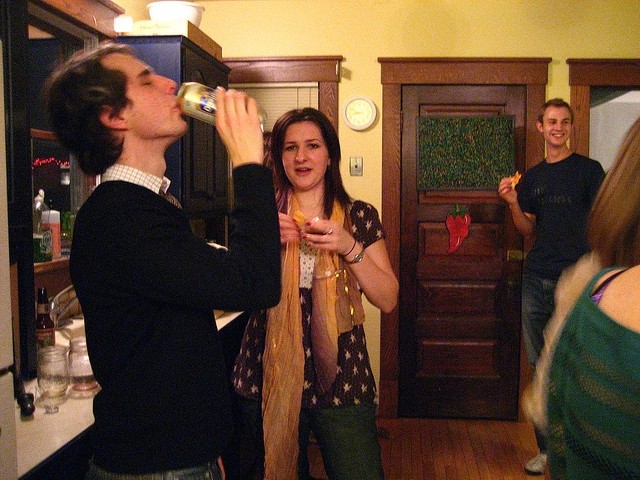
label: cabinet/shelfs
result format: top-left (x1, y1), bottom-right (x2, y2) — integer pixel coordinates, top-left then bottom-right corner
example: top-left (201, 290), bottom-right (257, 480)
top-left (116, 35), bottom-right (232, 216)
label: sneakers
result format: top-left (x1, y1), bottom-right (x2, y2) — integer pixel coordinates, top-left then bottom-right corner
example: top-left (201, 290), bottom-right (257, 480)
top-left (524, 453), bottom-right (547, 476)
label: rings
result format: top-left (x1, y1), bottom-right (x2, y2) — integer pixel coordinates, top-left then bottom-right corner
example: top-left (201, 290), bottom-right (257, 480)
top-left (327, 227), bottom-right (332, 234)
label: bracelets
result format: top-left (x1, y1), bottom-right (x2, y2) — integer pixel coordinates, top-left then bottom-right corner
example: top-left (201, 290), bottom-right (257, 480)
top-left (343, 239), bottom-right (358, 256)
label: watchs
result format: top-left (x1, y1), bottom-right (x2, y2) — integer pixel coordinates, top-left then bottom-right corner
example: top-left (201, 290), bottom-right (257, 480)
top-left (344, 246), bottom-right (366, 262)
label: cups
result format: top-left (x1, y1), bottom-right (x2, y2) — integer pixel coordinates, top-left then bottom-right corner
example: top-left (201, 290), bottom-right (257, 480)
top-left (68, 341), bottom-right (100, 400)
top-left (33, 190), bottom-right (53, 262)
top-left (36, 345), bottom-right (69, 407)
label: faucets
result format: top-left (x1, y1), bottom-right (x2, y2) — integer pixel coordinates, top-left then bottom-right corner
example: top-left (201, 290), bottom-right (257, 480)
top-left (49, 284), bottom-right (77, 327)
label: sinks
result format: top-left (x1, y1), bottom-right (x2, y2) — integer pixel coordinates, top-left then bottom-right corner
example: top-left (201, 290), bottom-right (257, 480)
top-left (54, 314), bottom-right (87, 347)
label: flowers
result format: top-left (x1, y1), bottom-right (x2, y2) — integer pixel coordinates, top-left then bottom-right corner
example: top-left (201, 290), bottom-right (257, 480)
top-left (31, 156), bottom-right (71, 192)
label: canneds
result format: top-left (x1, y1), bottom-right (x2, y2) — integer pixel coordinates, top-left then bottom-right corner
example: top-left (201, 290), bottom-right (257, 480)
top-left (176, 81), bottom-right (268, 134)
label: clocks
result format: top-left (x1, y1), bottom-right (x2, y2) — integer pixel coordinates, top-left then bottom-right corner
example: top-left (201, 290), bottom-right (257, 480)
top-left (343, 94), bottom-right (377, 131)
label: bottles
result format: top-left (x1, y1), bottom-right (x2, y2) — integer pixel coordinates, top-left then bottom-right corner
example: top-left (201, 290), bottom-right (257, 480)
top-left (31, 287), bottom-right (54, 384)
top-left (42, 210), bottom-right (61, 258)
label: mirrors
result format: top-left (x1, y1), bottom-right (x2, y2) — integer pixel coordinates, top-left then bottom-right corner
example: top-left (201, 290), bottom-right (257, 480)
top-left (13, 0), bottom-right (96, 274)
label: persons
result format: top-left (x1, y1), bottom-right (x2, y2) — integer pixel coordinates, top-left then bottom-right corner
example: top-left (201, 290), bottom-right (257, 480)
top-left (232, 108), bottom-right (400, 479)
top-left (544, 118), bottom-right (639, 479)
top-left (43, 41), bottom-right (283, 480)
top-left (497, 97), bottom-right (606, 475)
